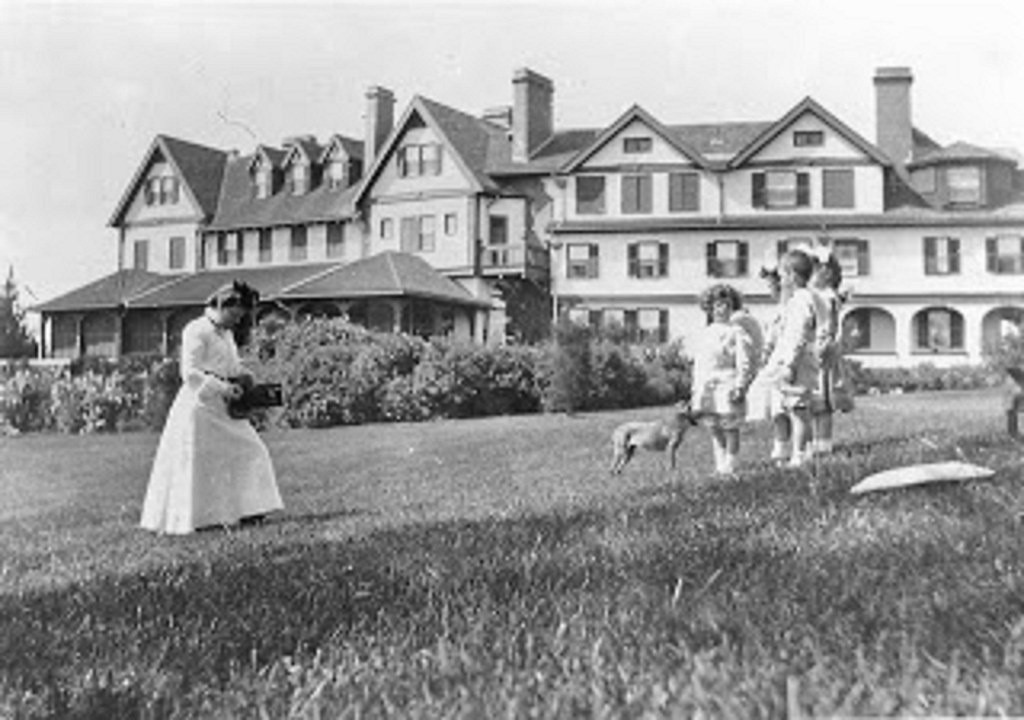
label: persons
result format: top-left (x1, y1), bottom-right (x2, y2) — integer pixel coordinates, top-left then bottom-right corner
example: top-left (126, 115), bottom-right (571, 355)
top-left (140, 275), bottom-right (287, 537)
top-left (690, 249), bottom-right (853, 475)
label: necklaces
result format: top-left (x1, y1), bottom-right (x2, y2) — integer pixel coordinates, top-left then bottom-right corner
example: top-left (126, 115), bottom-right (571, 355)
top-left (206, 316), bottom-right (223, 330)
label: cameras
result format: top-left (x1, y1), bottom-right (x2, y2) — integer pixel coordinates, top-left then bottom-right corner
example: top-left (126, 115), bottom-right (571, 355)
top-left (228, 374), bottom-right (283, 419)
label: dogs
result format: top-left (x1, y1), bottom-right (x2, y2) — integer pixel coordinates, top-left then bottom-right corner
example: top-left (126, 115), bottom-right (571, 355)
top-left (604, 405), bottom-right (698, 477)
